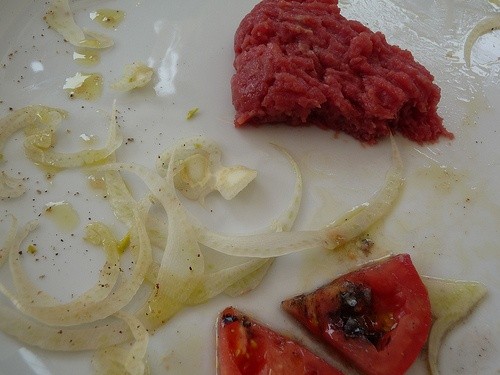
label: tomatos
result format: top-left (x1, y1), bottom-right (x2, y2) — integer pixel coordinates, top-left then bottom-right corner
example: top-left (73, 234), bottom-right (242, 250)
top-left (216, 254), bottom-right (433, 375)
